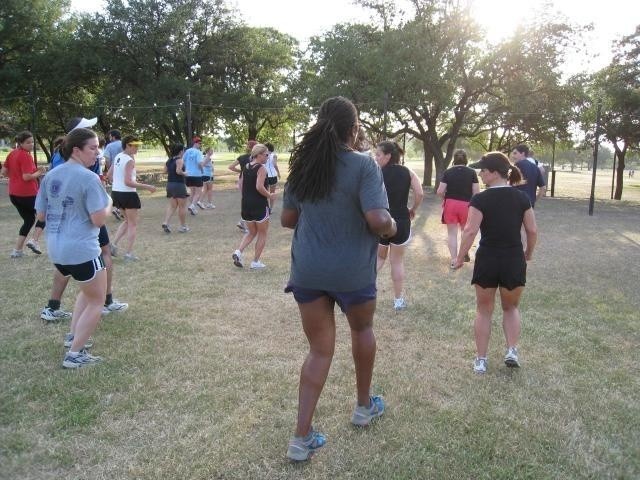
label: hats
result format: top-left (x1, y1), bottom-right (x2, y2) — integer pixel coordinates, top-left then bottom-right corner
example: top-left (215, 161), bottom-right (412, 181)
top-left (67, 117), bottom-right (97, 134)
top-left (127, 140), bottom-right (144, 145)
top-left (251, 144), bottom-right (268, 158)
top-left (468, 154), bottom-right (509, 172)
top-left (193, 136), bottom-right (201, 142)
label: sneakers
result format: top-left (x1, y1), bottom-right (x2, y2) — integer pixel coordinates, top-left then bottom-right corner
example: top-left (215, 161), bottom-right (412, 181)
top-left (110, 242), bottom-right (119, 257)
top-left (237, 221), bottom-right (249, 233)
top-left (393, 297), bottom-right (407, 310)
top-left (62, 332), bottom-right (102, 368)
top-left (350, 395), bottom-right (384, 426)
top-left (177, 224), bottom-right (190, 232)
top-left (122, 251), bottom-right (139, 261)
top-left (162, 223), bottom-right (171, 232)
top-left (250, 259), bottom-right (266, 269)
top-left (10, 248), bottom-right (28, 258)
top-left (40, 305), bottom-right (72, 320)
top-left (451, 252), bottom-right (470, 269)
top-left (26, 238), bottom-right (42, 254)
top-left (188, 200), bottom-right (216, 215)
top-left (504, 347), bottom-right (520, 367)
top-left (232, 249), bottom-right (244, 268)
top-left (112, 208), bottom-right (124, 220)
top-left (472, 355), bottom-right (487, 374)
top-left (102, 302), bottom-right (128, 313)
top-left (286, 426), bottom-right (326, 461)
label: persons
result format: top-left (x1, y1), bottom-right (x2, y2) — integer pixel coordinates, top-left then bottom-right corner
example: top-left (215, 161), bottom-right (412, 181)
top-left (284, 122), bottom-right (367, 294)
top-left (163, 140), bottom-right (280, 268)
top-left (279, 95), bottom-right (395, 461)
top-left (4, 122), bottom-right (157, 366)
top-left (373, 141), bottom-right (423, 308)
top-left (509, 145), bottom-right (545, 223)
top-left (435, 150), bottom-right (481, 267)
top-left (528, 149), bottom-right (545, 174)
top-left (454, 152), bottom-right (537, 373)
